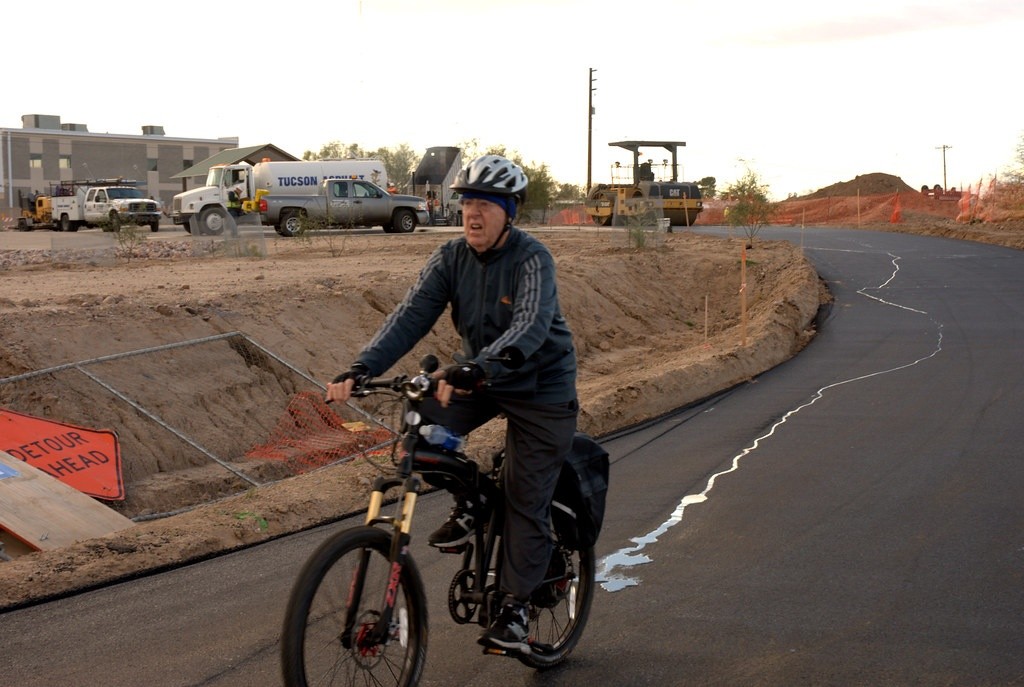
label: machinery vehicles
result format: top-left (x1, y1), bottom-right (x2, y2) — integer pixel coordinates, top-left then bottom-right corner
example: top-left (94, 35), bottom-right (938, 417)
top-left (19, 189), bottom-right (52, 230)
top-left (587, 140), bottom-right (704, 227)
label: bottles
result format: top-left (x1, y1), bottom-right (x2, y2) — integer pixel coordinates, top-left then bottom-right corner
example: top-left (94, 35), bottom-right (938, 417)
top-left (419, 424), bottom-right (465, 451)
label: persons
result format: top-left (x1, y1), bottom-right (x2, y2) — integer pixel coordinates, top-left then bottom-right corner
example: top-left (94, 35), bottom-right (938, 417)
top-left (324, 153), bottom-right (580, 656)
top-left (226, 184), bottom-right (250, 238)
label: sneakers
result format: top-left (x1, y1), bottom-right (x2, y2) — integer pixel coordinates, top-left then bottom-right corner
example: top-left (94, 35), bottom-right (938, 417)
top-left (426, 502), bottom-right (490, 547)
top-left (477, 602), bottom-right (530, 649)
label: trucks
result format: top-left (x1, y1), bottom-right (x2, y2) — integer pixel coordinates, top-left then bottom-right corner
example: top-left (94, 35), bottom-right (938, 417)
top-left (168, 157), bottom-right (388, 236)
top-left (52, 177), bottom-right (163, 232)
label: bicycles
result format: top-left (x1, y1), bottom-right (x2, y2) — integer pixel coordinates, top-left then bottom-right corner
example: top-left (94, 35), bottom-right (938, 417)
top-left (281, 353), bottom-right (595, 687)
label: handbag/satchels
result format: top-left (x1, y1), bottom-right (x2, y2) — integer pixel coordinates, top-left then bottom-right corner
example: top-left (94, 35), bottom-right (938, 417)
top-left (549, 437), bottom-right (609, 551)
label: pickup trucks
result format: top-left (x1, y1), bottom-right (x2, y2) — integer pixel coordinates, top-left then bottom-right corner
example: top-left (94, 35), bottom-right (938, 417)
top-left (259, 176), bottom-right (430, 237)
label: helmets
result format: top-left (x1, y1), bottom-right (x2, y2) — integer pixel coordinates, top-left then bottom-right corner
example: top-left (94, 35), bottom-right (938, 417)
top-left (449, 155), bottom-right (529, 206)
